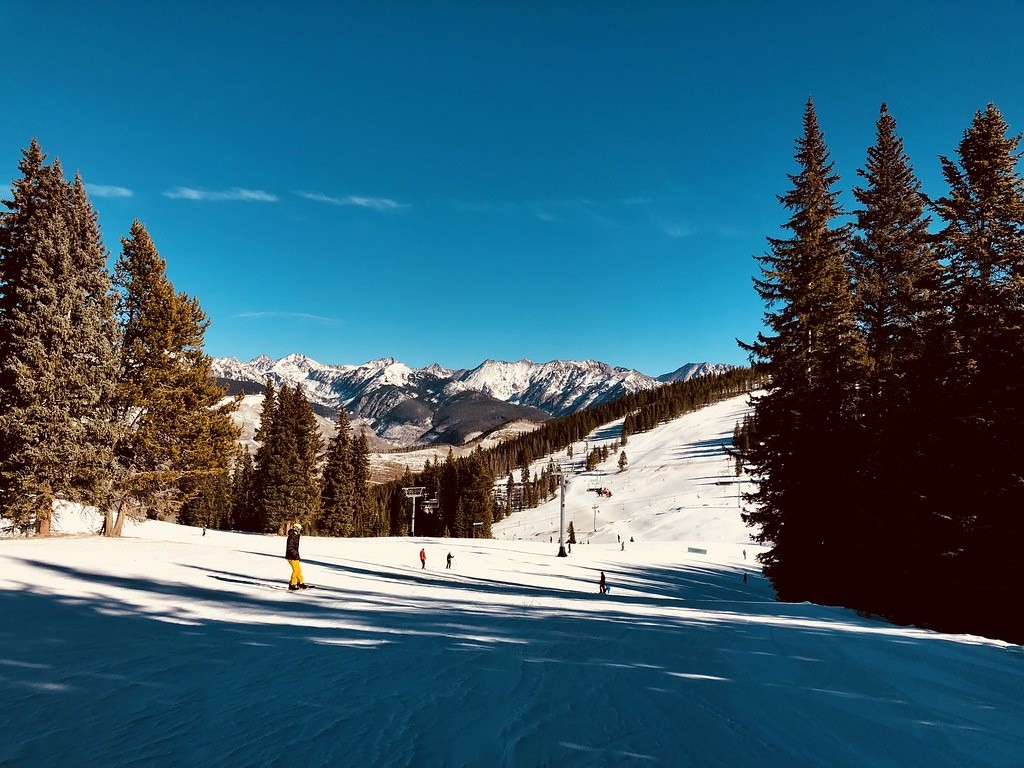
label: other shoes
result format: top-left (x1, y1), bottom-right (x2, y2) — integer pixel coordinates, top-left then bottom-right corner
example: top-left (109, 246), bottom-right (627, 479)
top-left (297, 583), bottom-right (307, 589)
top-left (289, 585), bottom-right (299, 590)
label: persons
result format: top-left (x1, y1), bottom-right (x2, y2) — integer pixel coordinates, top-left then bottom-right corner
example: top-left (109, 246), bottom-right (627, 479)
top-left (742, 573), bottom-right (748, 583)
top-left (549, 536), bottom-right (553, 544)
top-left (617, 534), bottom-right (620, 543)
top-left (597, 487), bottom-right (613, 498)
top-left (446, 551), bottom-right (454, 569)
top-left (597, 571), bottom-right (606, 595)
top-left (742, 549), bottom-right (748, 560)
top-left (621, 541), bottom-right (624, 551)
top-left (567, 542), bottom-right (571, 553)
top-left (419, 547), bottom-right (427, 571)
top-left (284, 523), bottom-right (311, 592)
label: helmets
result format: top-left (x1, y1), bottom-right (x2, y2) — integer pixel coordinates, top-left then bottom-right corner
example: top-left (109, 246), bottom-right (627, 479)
top-left (293, 523), bottom-right (304, 532)
